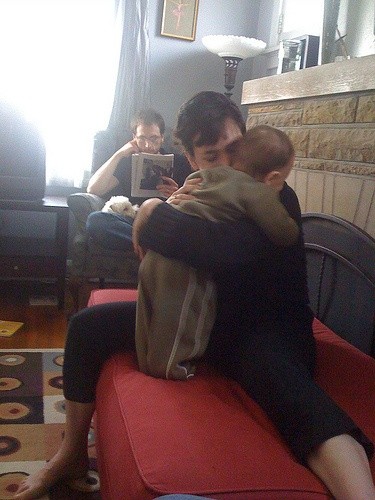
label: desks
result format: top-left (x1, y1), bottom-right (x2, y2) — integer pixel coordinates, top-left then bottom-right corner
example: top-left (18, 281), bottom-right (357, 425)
top-left (0, 197), bottom-right (70, 312)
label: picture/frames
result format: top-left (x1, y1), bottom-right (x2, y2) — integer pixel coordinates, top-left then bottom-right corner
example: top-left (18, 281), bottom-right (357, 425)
top-left (160, 0), bottom-right (199, 41)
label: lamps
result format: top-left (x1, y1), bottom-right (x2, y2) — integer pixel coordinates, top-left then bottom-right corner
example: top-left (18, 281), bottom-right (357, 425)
top-left (202, 34), bottom-right (267, 100)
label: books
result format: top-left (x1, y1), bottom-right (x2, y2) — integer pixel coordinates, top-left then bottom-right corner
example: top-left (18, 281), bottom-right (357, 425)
top-left (132, 153), bottom-right (174, 197)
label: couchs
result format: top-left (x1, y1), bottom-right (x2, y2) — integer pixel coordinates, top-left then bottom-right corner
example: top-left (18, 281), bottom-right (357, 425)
top-left (0, 104), bottom-right (46, 200)
top-left (68, 127), bottom-right (181, 289)
top-left (88, 212), bottom-right (375, 500)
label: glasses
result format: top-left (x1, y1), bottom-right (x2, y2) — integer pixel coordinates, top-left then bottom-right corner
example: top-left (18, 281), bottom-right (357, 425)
top-left (135, 135), bottom-right (161, 142)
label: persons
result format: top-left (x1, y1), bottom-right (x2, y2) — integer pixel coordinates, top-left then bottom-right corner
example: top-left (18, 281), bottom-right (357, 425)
top-left (135, 124), bottom-right (299, 380)
top-left (86, 108), bottom-right (192, 253)
top-left (171, 0), bottom-right (192, 30)
top-left (14, 92), bottom-right (375, 500)
